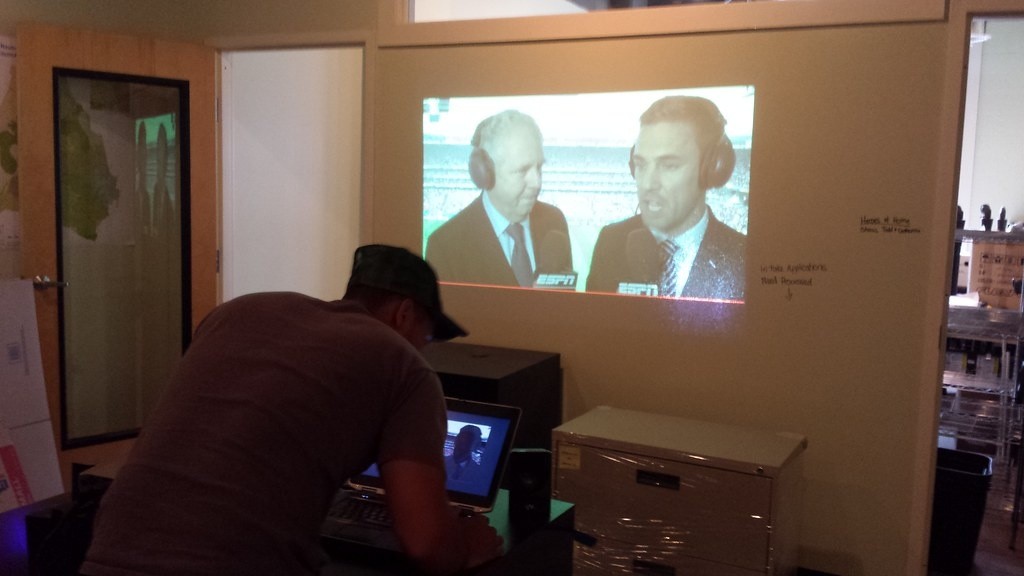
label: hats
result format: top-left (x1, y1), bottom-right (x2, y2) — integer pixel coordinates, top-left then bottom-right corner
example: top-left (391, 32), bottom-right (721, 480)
top-left (354, 244), bottom-right (468, 340)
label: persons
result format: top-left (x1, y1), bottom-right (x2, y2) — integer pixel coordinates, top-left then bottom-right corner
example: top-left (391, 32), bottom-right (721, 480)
top-left (445, 425), bottom-right (482, 492)
top-left (78, 243), bottom-right (505, 576)
top-left (426, 110), bottom-right (572, 288)
top-left (587, 95), bottom-right (744, 302)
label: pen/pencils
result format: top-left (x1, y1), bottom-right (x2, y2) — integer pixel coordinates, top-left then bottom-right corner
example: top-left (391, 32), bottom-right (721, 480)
top-left (463, 509), bottom-right (478, 518)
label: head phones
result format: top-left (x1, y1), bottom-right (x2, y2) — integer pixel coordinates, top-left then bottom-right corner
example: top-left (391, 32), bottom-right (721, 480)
top-left (629, 122), bottom-right (736, 191)
top-left (469, 119), bottom-right (495, 191)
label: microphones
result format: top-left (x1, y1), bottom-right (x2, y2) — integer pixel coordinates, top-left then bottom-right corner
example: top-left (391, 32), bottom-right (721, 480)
top-left (617, 229), bottom-right (663, 296)
top-left (531, 230), bottom-right (578, 290)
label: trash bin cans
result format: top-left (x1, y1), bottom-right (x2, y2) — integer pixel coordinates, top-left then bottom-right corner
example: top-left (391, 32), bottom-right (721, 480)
top-left (928, 444), bottom-right (993, 575)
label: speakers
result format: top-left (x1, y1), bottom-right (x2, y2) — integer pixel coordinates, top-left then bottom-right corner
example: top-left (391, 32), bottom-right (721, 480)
top-left (509, 447), bottom-right (553, 533)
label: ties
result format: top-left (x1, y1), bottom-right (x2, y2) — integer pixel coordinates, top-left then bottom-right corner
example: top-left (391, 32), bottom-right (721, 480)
top-left (658, 239), bottom-right (681, 298)
top-left (506, 223), bottom-right (535, 287)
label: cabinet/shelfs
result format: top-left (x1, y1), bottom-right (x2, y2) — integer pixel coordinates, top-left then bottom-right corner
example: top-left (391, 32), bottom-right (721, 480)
top-left (938, 306), bottom-right (1024, 465)
top-left (551, 406), bottom-right (808, 576)
top-left (426, 342), bottom-right (559, 541)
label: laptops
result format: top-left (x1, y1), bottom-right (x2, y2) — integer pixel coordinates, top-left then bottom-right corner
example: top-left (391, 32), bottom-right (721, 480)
top-left (318, 397), bottom-right (523, 553)
